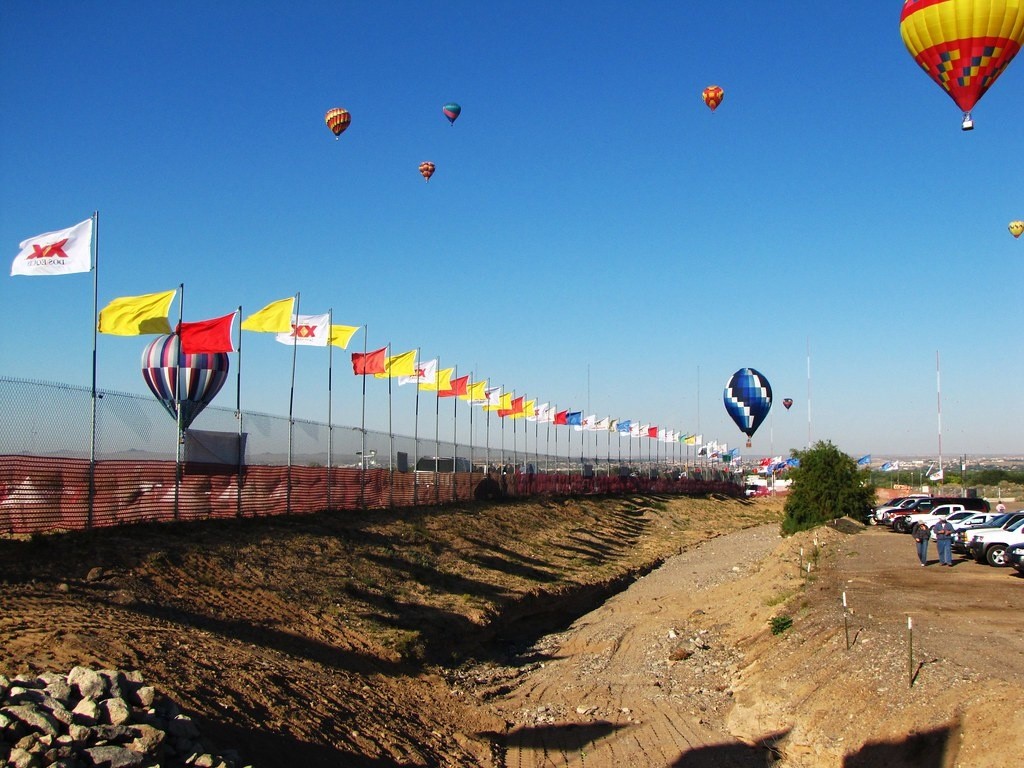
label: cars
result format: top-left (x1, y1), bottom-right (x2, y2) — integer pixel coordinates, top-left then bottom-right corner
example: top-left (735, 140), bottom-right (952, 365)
top-left (865, 494), bottom-right (929, 526)
top-left (930, 511), bottom-right (1024, 576)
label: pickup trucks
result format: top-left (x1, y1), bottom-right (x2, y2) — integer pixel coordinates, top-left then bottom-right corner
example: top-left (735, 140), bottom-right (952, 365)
top-left (904, 505), bottom-right (965, 532)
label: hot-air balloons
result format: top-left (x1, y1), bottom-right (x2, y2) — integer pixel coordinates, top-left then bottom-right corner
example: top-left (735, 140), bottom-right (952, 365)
top-left (899, 0), bottom-right (1024, 131)
top-left (702, 85), bottom-right (725, 114)
top-left (783, 398), bottom-right (793, 412)
top-left (1008, 220), bottom-right (1024, 240)
top-left (722, 367), bottom-right (773, 449)
top-left (325, 108), bottom-right (352, 141)
top-left (443, 103), bottom-right (461, 127)
top-left (419, 162), bottom-right (435, 184)
top-left (141, 332), bottom-right (230, 444)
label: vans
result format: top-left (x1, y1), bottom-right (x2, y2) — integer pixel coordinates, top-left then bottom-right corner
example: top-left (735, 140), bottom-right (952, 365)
top-left (412, 455), bottom-right (477, 487)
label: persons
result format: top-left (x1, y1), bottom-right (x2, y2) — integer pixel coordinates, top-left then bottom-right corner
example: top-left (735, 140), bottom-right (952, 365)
top-left (933, 516), bottom-right (955, 566)
top-left (995, 500), bottom-right (1005, 513)
top-left (911, 520), bottom-right (931, 567)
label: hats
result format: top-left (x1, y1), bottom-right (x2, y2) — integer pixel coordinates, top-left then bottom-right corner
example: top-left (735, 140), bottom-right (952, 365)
top-left (918, 520), bottom-right (924, 526)
top-left (940, 517), bottom-right (946, 521)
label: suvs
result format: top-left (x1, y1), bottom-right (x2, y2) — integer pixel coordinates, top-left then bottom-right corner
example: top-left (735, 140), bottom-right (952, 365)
top-left (882, 497), bottom-right (990, 532)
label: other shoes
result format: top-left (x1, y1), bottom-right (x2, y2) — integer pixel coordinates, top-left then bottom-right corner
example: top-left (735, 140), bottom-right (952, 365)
top-left (940, 562), bottom-right (944, 566)
top-left (948, 562), bottom-right (952, 566)
top-left (921, 563), bottom-right (925, 567)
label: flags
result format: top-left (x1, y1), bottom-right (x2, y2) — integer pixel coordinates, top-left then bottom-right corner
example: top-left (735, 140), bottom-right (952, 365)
top-left (274, 311), bottom-right (743, 468)
top-left (238, 297), bottom-right (296, 333)
top-left (175, 313), bottom-right (236, 355)
top-left (10, 218), bottom-right (92, 276)
top-left (97, 288), bottom-right (174, 334)
top-left (752, 456), bottom-right (801, 474)
top-left (881, 461), bottom-right (944, 481)
top-left (858, 455), bottom-right (871, 466)
